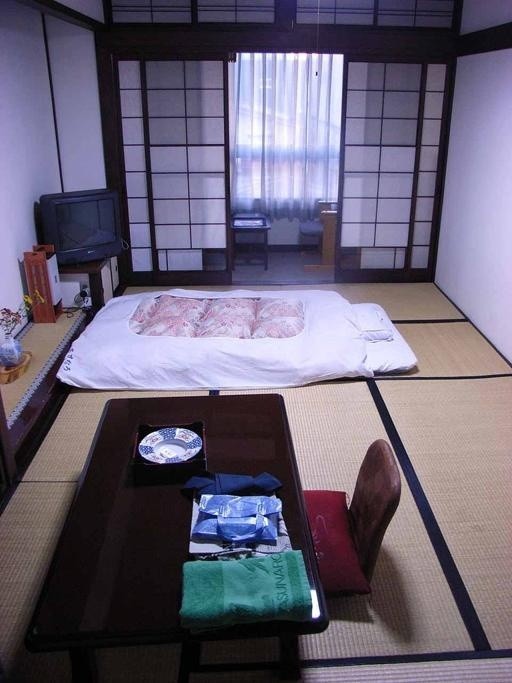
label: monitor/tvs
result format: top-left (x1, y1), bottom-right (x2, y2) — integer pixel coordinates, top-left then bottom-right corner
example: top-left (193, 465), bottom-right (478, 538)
top-left (39, 188), bottom-right (122, 266)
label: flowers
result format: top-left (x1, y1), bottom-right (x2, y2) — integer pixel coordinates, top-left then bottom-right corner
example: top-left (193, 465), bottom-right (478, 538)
top-left (1, 286), bottom-right (46, 335)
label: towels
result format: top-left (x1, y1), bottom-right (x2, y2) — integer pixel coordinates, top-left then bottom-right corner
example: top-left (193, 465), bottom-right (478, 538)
top-left (172, 542), bottom-right (313, 637)
top-left (178, 462), bottom-right (289, 500)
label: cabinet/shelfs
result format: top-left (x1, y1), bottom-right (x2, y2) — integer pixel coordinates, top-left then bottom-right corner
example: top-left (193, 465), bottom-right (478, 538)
top-left (54, 253), bottom-right (125, 327)
top-left (0, 303), bottom-right (97, 505)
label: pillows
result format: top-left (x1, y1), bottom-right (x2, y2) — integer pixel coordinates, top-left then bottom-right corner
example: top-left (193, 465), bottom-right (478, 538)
top-left (346, 300), bottom-right (396, 343)
top-left (302, 487), bottom-right (372, 599)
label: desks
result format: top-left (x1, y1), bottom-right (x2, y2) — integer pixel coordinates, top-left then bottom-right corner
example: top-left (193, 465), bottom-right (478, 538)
top-left (20, 386), bottom-right (333, 682)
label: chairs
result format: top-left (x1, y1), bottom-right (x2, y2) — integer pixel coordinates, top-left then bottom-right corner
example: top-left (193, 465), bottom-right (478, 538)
top-left (299, 435), bottom-right (403, 601)
top-left (297, 197), bottom-right (341, 274)
top-left (227, 207), bottom-right (273, 273)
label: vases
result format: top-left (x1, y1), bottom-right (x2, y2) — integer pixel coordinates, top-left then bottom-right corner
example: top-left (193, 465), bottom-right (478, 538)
top-left (0, 331), bottom-right (27, 369)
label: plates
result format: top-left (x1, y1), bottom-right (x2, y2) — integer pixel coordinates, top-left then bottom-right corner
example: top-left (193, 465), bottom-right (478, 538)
top-left (138, 427), bottom-right (202, 464)
